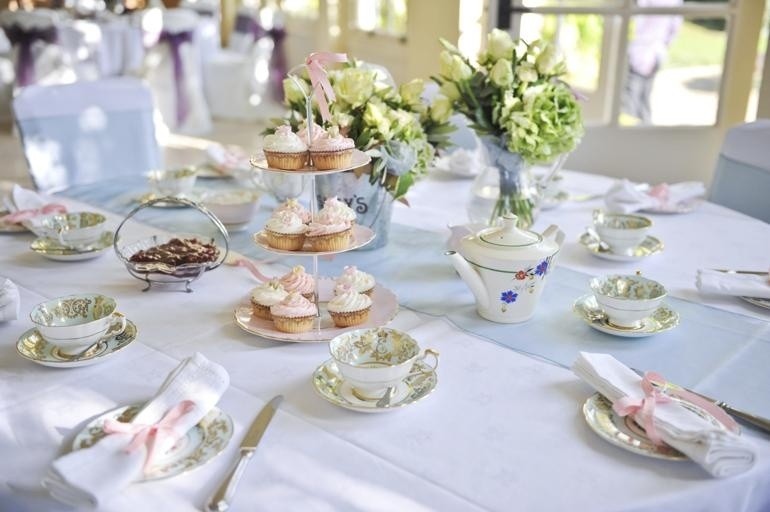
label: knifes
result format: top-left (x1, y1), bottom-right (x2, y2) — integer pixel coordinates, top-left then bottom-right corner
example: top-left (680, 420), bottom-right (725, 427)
top-left (629, 365), bottom-right (768, 437)
top-left (207, 394), bottom-right (286, 511)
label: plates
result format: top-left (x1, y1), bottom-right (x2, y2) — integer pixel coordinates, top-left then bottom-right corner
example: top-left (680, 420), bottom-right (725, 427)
top-left (15, 316), bottom-right (135, 370)
top-left (27, 230), bottom-right (122, 263)
top-left (110, 195), bottom-right (230, 287)
top-left (718, 268), bottom-right (769, 308)
top-left (71, 401), bottom-right (234, 484)
top-left (626, 181), bottom-right (702, 216)
top-left (582, 386), bottom-right (732, 470)
top-left (312, 347), bottom-right (440, 414)
top-left (578, 229), bottom-right (664, 260)
top-left (573, 290), bottom-right (681, 338)
top-left (466, 178), bottom-right (531, 208)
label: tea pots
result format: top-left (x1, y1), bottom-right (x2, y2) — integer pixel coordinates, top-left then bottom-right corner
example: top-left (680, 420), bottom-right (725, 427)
top-left (447, 214), bottom-right (569, 326)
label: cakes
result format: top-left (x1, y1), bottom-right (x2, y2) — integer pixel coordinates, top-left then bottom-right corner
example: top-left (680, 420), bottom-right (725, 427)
top-left (264, 211), bottom-right (309, 251)
top-left (326, 287), bottom-right (372, 327)
top-left (269, 289), bottom-right (317, 332)
top-left (317, 196), bottom-right (356, 235)
top-left (272, 197), bottom-right (311, 226)
top-left (263, 124), bottom-right (307, 170)
top-left (309, 126), bottom-right (356, 170)
top-left (278, 265), bottom-right (315, 302)
top-left (295, 118), bottom-right (326, 146)
top-left (333, 265), bottom-right (376, 299)
top-left (306, 209), bottom-right (352, 252)
top-left (249, 277), bottom-right (290, 320)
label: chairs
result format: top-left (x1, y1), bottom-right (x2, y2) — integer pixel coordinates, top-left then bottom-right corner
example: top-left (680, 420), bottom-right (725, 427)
top-left (8, 77), bottom-right (162, 193)
top-left (701, 116), bottom-right (770, 227)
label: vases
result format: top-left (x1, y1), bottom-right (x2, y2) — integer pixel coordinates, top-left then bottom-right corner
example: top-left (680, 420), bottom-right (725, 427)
top-left (312, 170), bottom-right (393, 254)
top-left (479, 168), bottom-right (538, 232)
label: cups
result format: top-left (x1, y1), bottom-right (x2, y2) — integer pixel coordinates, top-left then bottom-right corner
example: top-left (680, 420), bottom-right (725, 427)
top-left (594, 209), bottom-right (651, 253)
top-left (204, 189), bottom-right (257, 236)
top-left (589, 271), bottom-right (670, 328)
top-left (326, 324), bottom-right (440, 398)
top-left (43, 209), bottom-right (107, 254)
top-left (29, 292), bottom-right (129, 356)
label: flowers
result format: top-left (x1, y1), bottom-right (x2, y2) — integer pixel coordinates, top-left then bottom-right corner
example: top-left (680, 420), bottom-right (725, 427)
top-left (260, 55), bottom-right (460, 209)
top-left (423, 23), bottom-right (587, 232)
